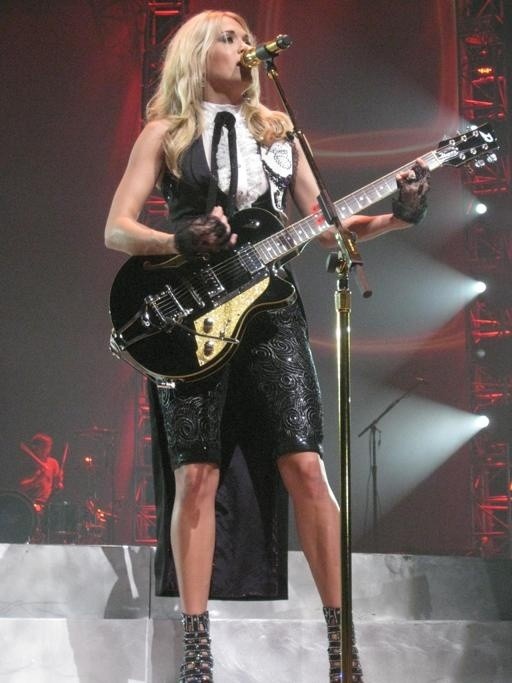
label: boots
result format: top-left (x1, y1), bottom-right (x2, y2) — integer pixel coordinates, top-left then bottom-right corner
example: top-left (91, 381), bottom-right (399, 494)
top-left (323, 605), bottom-right (364, 683)
top-left (182, 610), bottom-right (215, 682)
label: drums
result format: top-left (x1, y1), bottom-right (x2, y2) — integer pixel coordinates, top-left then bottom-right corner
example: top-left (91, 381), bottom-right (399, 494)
top-left (43, 500), bottom-right (78, 537)
top-left (0, 490), bottom-right (36, 544)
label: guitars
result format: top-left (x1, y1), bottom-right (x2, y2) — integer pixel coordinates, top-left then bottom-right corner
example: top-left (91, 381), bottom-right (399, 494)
top-left (109, 122), bottom-right (501, 389)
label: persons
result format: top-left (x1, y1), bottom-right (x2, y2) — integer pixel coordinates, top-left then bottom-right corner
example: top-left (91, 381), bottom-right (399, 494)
top-left (102, 7), bottom-right (433, 683)
top-left (19, 431), bottom-right (65, 502)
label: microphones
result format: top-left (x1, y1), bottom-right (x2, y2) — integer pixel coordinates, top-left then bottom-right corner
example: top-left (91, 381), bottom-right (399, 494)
top-left (240, 35), bottom-right (290, 68)
